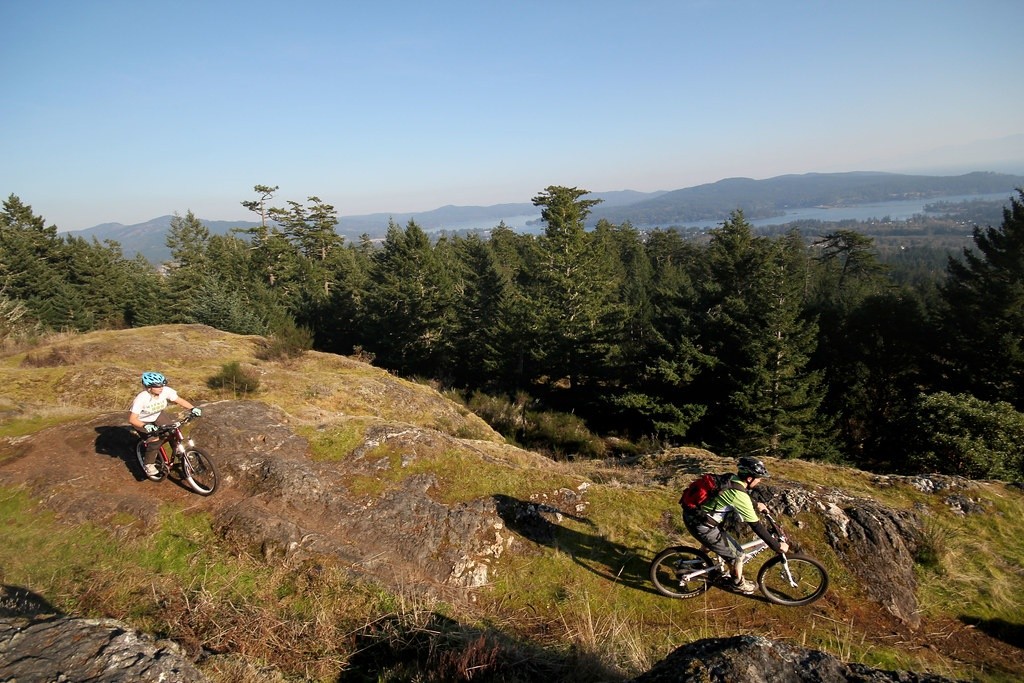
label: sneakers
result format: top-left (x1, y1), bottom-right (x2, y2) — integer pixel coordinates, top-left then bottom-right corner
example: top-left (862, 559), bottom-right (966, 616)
top-left (731, 576), bottom-right (756, 591)
top-left (143, 463), bottom-right (159, 476)
top-left (176, 454), bottom-right (194, 459)
top-left (697, 552), bottom-right (712, 560)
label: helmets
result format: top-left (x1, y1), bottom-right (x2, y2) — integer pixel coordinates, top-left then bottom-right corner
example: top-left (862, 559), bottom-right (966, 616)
top-left (737, 456), bottom-right (771, 478)
top-left (142, 372), bottom-right (167, 388)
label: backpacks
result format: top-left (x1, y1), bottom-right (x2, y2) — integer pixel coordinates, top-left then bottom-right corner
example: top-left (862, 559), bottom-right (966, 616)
top-left (679, 473), bottom-right (748, 513)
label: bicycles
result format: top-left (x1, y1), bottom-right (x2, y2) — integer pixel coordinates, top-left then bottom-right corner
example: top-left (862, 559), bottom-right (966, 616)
top-left (135, 414), bottom-right (220, 497)
top-left (651, 509), bottom-right (830, 607)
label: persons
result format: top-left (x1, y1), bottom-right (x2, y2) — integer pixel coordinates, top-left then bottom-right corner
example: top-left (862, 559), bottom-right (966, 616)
top-left (682, 457), bottom-right (789, 594)
top-left (130, 372), bottom-right (201, 476)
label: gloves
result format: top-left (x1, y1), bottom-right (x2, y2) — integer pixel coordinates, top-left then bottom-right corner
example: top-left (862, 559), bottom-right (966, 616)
top-left (191, 407), bottom-right (201, 417)
top-left (143, 424), bottom-right (158, 433)
